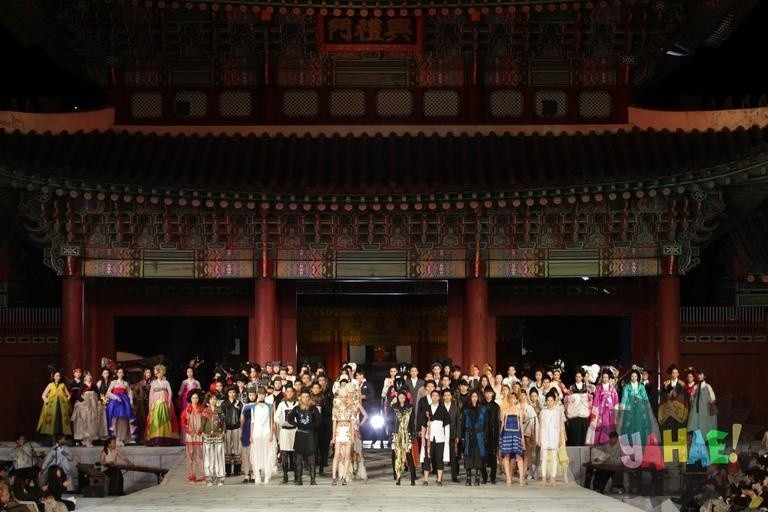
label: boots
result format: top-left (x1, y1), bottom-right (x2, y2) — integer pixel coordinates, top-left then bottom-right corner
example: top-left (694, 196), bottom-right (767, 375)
top-left (282, 461), bottom-right (317, 485)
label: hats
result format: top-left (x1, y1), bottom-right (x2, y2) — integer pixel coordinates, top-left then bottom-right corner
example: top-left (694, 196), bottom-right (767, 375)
top-left (432, 362), bottom-right (442, 369)
top-left (257, 388), bottom-right (265, 393)
top-left (247, 387), bottom-right (256, 393)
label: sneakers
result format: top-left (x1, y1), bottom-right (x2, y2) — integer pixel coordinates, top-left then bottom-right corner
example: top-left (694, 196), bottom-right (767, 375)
top-left (242, 475), bottom-right (269, 484)
top-left (83, 440), bottom-right (94, 448)
top-left (116, 440), bottom-right (125, 447)
top-left (189, 475), bottom-right (224, 487)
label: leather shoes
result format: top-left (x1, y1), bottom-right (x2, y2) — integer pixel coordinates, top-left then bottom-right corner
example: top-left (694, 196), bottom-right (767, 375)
top-left (452, 478), bottom-right (497, 486)
top-left (332, 477), bottom-right (348, 485)
top-left (396, 480), bottom-right (443, 486)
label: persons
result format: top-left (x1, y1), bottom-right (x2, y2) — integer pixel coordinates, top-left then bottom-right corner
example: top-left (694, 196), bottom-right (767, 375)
top-left (1, 356), bottom-right (768, 512)
